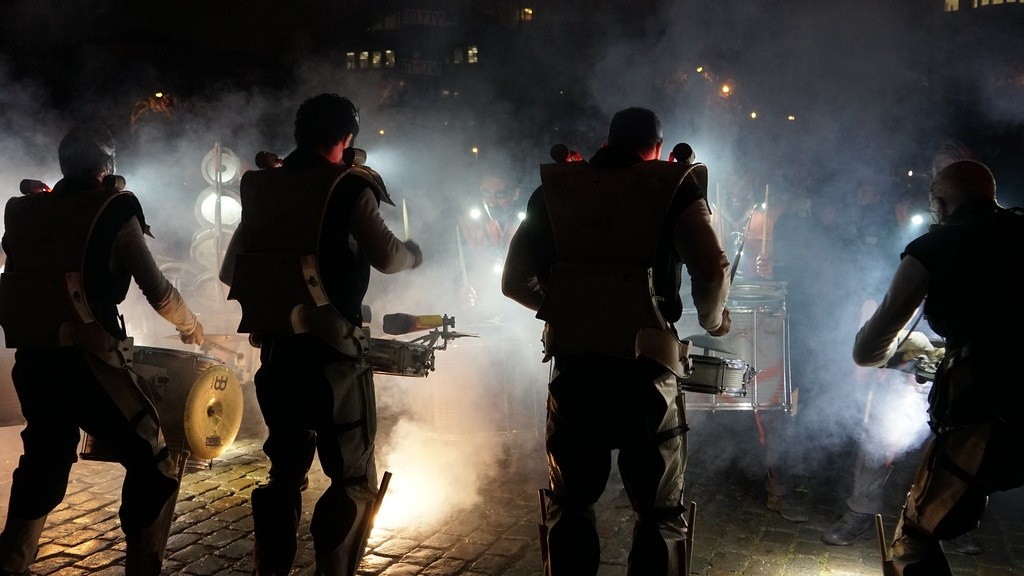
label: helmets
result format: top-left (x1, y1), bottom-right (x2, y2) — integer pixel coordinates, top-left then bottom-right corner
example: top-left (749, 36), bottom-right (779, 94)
top-left (929, 159), bottom-right (996, 215)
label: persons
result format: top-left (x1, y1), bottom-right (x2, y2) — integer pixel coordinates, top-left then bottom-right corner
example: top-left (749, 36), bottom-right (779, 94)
top-left (218, 92), bottom-right (425, 576)
top-left (0, 119), bottom-right (205, 576)
top-left (853, 160), bottom-right (1024, 576)
top-left (501, 106), bottom-right (733, 574)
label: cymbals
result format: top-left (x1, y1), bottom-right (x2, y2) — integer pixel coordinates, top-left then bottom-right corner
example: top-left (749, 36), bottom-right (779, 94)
top-left (184, 364), bottom-right (244, 461)
top-left (434, 331), bottom-right (479, 339)
top-left (682, 335), bottom-right (736, 356)
top-left (163, 332), bottom-right (249, 346)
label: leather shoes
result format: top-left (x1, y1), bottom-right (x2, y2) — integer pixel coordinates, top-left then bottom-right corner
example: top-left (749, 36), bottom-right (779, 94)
top-left (821, 507), bottom-right (877, 545)
top-left (948, 530), bottom-right (984, 554)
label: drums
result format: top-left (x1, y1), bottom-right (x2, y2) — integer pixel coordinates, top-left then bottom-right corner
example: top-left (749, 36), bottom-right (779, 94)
top-left (370, 338), bottom-right (430, 377)
top-left (680, 355), bottom-right (750, 397)
top-left (78, 344), bottom-right (223, 471)
